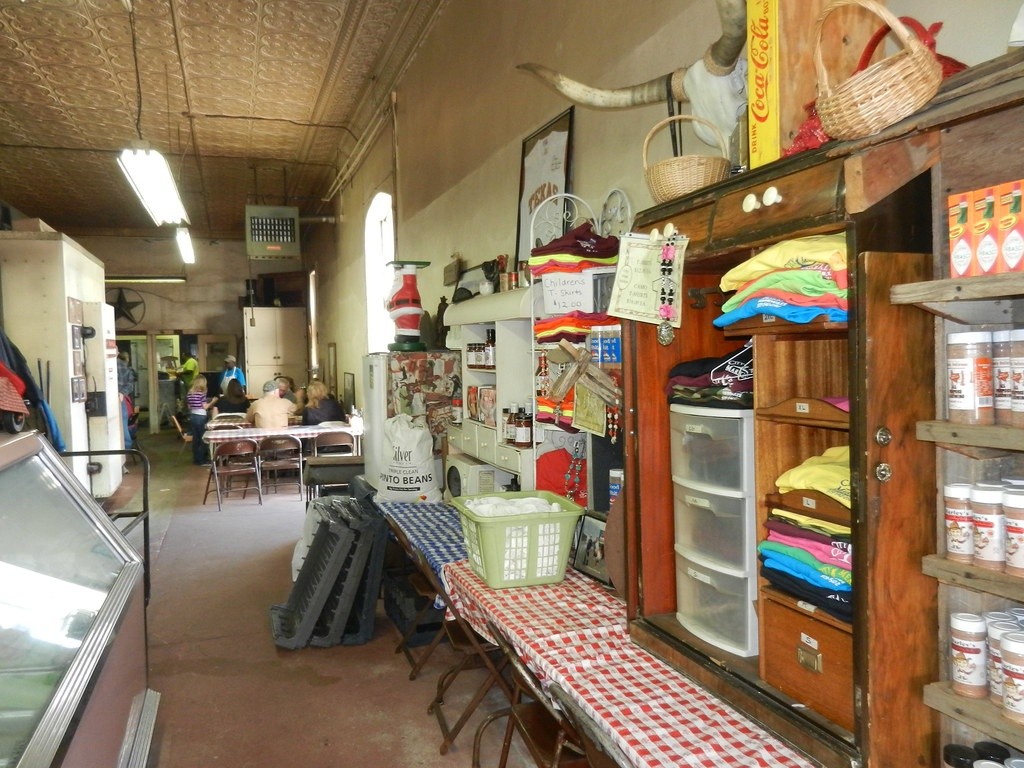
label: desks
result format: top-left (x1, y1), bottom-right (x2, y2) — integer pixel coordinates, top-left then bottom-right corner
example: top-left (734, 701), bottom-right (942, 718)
top-left (381, 502), bottom-right (472, 605)
top-left (538, 638), bottom-right (821, 768)
top-left (203, 426), bottom-right (364, 498)
top-left (442, 556), bottom-right (632, 679)
top-left (207, 415), bottom-right (304, 429)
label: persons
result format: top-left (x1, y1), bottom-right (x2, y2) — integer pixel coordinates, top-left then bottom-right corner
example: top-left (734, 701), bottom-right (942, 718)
top-left (115, 345), bottom-right (139, 450)
top-left (212, 378), bottom-right (250, 419)
top-left (217, 355), bottom-right (247, 397)
top-left (186, 374), bottom-right (219, 465)
top-left (275, 377), bottom-right (294, 402)
top-left (302, 380), bottom-right (351, 453)
top-left (246, 380), bottom-right (298, 455)
top-left (176, 351), bottom-right (199, 392)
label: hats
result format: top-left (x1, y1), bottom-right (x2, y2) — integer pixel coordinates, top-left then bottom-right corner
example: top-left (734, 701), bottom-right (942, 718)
top-left (263, 381), bottom-right (281, 392)
top-left (224, 355), bottom-right (236, 363)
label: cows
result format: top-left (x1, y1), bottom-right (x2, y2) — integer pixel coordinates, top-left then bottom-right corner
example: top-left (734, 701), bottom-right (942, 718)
top-left (515, 0), bottom-right (748, 173)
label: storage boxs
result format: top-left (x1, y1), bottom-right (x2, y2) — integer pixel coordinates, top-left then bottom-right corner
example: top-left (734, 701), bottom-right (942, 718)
top-left (450, 488), bottom-right (586, 589)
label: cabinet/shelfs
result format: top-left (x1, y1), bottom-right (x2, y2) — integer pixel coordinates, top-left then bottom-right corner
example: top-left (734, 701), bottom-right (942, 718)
top-left (242, 306), bottom-right (308, 395)
top-left (445, 319), bottom-right (534, 489)
top-left (629, 104), bottom-right (1024, 766)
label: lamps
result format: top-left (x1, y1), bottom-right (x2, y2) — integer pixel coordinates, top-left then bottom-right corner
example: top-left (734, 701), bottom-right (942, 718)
top-left (115, 139), bottom-right (192, 227)
top-left (174, 227), bottom-right (196, 264)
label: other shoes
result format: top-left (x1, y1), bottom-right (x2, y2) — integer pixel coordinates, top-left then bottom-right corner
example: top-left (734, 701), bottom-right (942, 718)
top-left (201, 460), bottom-right (212, 466)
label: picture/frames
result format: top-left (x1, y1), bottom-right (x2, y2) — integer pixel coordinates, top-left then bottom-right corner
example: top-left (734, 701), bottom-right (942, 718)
top-left (343, 372), bottom-right (355, 414)
top-left (514, 104), bottom-right (574, 273)
top-left (328, 342), bottom-right (337, 400)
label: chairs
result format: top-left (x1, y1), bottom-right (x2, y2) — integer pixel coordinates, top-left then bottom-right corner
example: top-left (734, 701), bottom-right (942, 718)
top-left (372, 515), bottom-right (619, 768)
top-left (167, 415), bottom-right (354, 513)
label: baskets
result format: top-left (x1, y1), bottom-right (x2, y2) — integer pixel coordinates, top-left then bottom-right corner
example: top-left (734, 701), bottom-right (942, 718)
top-left (810, 1), bottom-right (945, 143)
top-left (448, 490), bottom-right (586, 589)
top-left (641, 116), bottom-right (729, 205)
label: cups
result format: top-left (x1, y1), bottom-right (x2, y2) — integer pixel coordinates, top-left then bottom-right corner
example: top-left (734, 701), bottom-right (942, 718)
top-left (478, 282), bottom-right (493, 295)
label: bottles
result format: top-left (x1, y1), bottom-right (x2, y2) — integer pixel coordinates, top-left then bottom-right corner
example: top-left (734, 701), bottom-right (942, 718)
top-left (941, 329), bottom-right (1024, 766)
top-left (465, 259), bottom-right (535, 449)
top-left (587, 319), bottom-right (623, 513)
